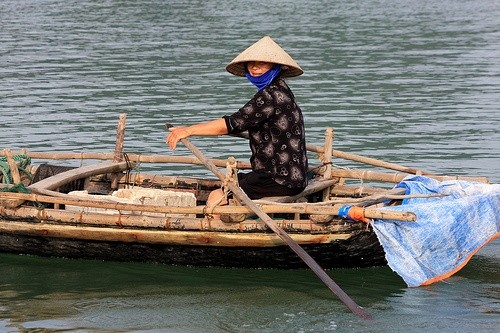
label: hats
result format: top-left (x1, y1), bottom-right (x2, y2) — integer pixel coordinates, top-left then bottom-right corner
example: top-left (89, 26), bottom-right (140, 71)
top-left (226, 36), bottom-right (304, 78)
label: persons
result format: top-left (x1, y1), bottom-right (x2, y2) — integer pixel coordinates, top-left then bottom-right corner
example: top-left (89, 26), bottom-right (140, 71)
top-left (165, 36), bottom-right (310, 219)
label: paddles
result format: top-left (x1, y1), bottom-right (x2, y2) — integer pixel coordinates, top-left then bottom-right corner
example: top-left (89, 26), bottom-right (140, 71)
top-left (227, 131), bottom-right (425, 175)
top-left (167, 123), bottom-right (378, 321)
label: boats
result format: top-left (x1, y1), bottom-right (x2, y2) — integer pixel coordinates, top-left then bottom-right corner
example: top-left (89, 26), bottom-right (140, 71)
top-left (0, 114), bottom-right (500, 271)
